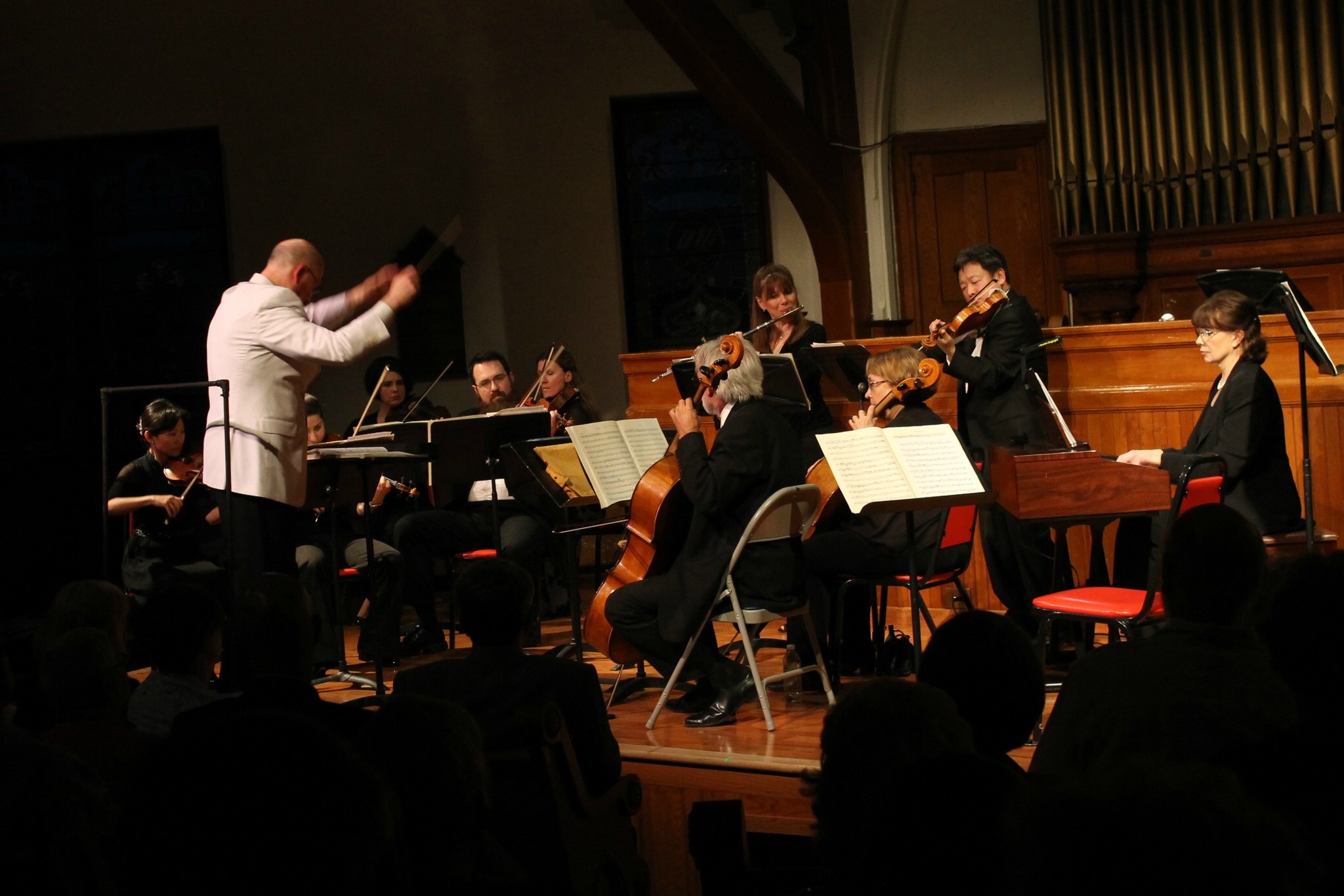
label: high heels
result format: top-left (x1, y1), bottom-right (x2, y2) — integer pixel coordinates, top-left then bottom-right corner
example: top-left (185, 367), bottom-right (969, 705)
top-left (768, 664), bottom-right (838, 691)
top-left (840, 641), bottom-right (875, 677)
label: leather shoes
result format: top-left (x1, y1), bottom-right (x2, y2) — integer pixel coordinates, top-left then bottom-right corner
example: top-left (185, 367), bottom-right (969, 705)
top-left (399, 623), bottom-right (449, 656)
top-left (355, 610), bottom-right (371, 624)
top-left (685, 669), bottom-right (762, 726)
top-left (356, 634), bottom-right (400, 667)
top-left (665, 680), bottom-right (716, 713)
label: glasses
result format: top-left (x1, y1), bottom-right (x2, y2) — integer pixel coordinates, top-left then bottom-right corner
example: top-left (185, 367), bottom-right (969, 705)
top-left (692, 377), bottom-right (703, 392)
top-left (1198, 329), bottom-right (1217, 342)
top-left (307, 267), bottom-right (324, 293)
top-left (869, 380), bottom-right (889, 391)
top-left (381, 380), bottom-right (403, 389)
top-left (474, 373), bottom-right (507, 390)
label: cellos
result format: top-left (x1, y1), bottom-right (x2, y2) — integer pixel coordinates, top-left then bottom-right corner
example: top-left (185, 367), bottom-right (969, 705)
top-left (731, 358), bottom-right (943, 634)
top-left (582, 334), bottom-right (743, 713)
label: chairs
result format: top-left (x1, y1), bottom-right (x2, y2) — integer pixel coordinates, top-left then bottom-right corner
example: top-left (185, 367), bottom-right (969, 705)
top-left (869, 445), bottom-right (986, 673)
top-left (449, 548), bottom-right (497, 649)
top-left (1024, 452), bottom-right (1227, 746)
top-left (645, 483), bottom-right (836, 731)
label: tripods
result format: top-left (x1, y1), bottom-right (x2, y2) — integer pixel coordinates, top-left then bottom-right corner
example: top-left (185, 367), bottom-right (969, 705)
top-left (569, 443), bottom-right (698, 708)
top-left (295, 451), bottom-right (391, 694)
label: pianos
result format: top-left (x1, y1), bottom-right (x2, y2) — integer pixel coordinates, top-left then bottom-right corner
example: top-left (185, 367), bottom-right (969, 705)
top-left (989, 441), bottom-right (1172, 670)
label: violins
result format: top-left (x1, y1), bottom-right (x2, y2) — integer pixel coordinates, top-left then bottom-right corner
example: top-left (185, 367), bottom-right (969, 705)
top-left (162, 384), bottom-right (580, 514)
top-left (921, 291), bottom-right (1005, 349)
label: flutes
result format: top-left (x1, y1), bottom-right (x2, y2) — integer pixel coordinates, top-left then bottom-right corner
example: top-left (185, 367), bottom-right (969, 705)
top-left (650, 304), bottom-right (805, 384)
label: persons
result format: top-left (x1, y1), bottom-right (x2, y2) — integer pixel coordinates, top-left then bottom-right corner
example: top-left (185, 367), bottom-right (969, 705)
top-left (108, 397), bottom-right (220, 589)
top-left (729, 263), bottom-right (835, 468)
top-left (798, 503), bottom-right (1344, 896)
top-left (766, 347), bottom-right (965, 693)
top-left (909, 242), bottom-right (1058, 644)
top-left (202, 242), bottom-right (419, 699)
top-left (1116, 289), bottom-right (1302, 535)
top-left (294, 348), bottom-right (603, 669)
top-left (0, 557), bottom-right (650, 896)
top-left (604, 335), bottom-right (804, 727)
